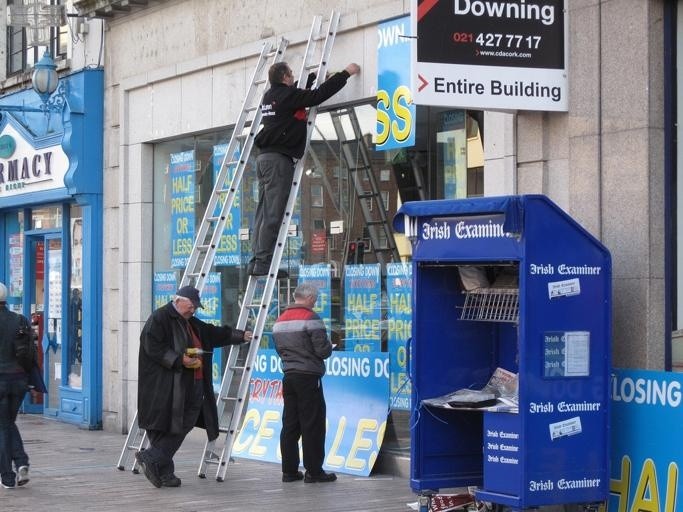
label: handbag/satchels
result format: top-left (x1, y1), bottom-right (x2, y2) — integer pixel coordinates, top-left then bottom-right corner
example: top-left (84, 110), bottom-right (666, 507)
top-left (13, 322), bottom-right (39, 373)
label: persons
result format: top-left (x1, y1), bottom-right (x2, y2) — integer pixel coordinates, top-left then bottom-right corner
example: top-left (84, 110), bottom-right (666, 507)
top-left (248, 61), bottom-right (358, 274)
top-left (134, 287), bottom-right (252, 488)
top-left (69, 288), bottom-right (81, 364)
top-left (0, 280), bottom-right (37, 489)
top-left (270, 283), bottom-right (337, 483)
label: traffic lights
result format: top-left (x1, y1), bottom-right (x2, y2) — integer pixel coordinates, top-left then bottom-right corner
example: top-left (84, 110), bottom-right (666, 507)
top-left (357, 241), bottom-right (364, 264)
top-left (347, 242), bottom-right (355, 265)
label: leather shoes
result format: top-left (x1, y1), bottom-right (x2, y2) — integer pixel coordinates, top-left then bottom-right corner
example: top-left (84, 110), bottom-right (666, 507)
top-left (281, 471), bottom-right (303, 483)
top-left (304, 471), bottom-right (338, 484)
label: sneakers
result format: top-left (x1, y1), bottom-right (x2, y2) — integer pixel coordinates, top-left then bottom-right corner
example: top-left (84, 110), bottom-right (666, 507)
top-left (247, 261), bottom-right (255, 275)
top-left (160, 472), bottom-right (181, 489)
top-left (16, 464), bottom-right (30, 487)
top-left (253, 260), bottom-right (288, 280)
top-left (134, 448), bottom-right (161, 488)
top-left (0, 471), bottom-right (16, 489)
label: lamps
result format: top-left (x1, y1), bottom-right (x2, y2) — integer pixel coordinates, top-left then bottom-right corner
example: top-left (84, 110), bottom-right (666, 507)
top-left (27, 44), bottom-right (72, 118)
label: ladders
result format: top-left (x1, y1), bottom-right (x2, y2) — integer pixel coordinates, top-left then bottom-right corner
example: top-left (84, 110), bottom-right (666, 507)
top-left (330, 105), bottom-right (402, 294)
top-left (117, 35), bottom-right (287, 474)
top-left (198, 9), bottom-right (340, 482)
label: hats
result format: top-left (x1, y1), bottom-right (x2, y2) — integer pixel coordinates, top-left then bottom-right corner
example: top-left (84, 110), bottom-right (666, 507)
top-left (176, 285), bottom-right (205, 310)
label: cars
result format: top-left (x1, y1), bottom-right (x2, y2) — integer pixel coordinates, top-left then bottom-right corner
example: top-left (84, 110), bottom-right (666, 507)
top-left (265, 303), bottom-right (388, 346)
